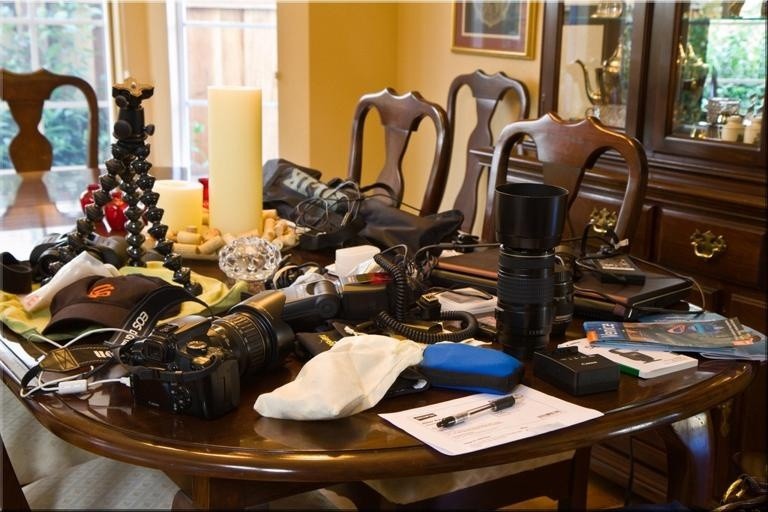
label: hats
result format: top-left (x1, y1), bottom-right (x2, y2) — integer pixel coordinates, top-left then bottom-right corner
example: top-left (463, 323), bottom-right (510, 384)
top-left (41, 274), bottom-right (180, 335)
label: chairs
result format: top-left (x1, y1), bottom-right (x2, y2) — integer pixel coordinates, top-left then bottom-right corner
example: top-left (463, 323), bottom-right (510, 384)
top-left (346, 69), bottom-right (651, 254)
top-left (0, 65), bottom-right (105, 169)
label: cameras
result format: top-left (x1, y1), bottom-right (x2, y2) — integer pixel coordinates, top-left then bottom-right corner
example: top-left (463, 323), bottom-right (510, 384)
top-left (29, 229), bottom-right (128, 286)
top-left (127, 290), bottom-right (296, 422)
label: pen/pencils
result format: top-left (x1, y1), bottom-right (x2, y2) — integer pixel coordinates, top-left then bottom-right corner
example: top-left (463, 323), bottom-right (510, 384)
top-left (437, 394), bottom-right (524, 428)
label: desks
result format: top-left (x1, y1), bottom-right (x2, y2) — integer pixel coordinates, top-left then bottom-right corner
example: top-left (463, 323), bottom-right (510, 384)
top-left (1, 167), bottom-right (753, 509)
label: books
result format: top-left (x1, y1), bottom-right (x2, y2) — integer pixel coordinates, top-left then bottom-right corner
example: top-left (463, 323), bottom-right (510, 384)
top-left (557, 311), bottom-right (768, 378)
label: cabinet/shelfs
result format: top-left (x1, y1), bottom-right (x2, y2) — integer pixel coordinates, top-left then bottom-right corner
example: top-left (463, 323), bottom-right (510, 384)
top-left (469, 2), bottom-right (767, 456)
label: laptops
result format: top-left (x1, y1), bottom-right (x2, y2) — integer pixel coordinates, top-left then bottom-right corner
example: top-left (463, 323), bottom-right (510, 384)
top-left (431, 247), bottom-right (693, 321)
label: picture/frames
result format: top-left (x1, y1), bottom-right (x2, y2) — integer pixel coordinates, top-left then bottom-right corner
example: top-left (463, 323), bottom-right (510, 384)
top-left (448, 1), bottom-right (540, 65)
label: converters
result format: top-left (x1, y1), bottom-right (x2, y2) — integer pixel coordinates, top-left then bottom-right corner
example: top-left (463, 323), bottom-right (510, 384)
top-left (591, 254), bottom-right (645, 287)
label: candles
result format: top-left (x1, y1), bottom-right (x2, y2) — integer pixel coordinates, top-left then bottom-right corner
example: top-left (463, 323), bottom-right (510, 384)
top-left (149, 176), bottom-right (203, 239)
top-left (203, 84), bottom-right (266, 237)
